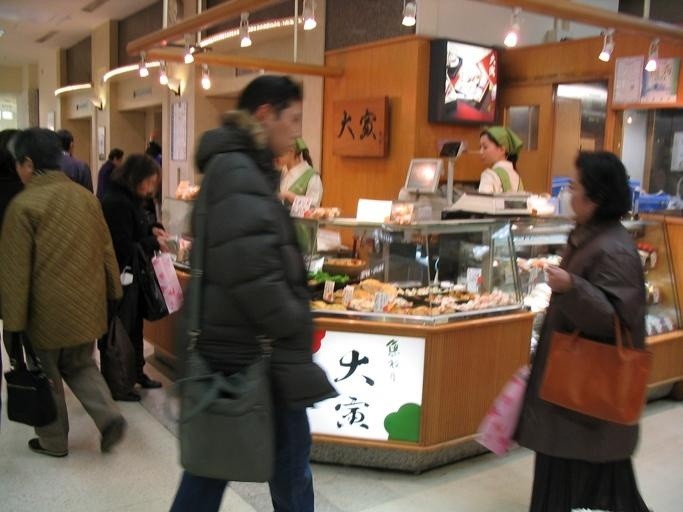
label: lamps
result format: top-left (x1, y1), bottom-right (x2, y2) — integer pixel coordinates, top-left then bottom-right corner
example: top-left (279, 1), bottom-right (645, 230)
top-left (91, 99), bottom-right (102, 110)
top-left (137, 0), bottom-right (417, 91)
top-left (500, 4), bottom-right (664, 74)
top-left (166, 78), bottom-right (182, 98)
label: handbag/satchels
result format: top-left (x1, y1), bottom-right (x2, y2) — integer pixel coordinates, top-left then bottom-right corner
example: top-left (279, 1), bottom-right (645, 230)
top-left (474, 365), bottom-right (534, 455)
top-left (165, 342), bottom-right (276, 482)
top-left (100, 298), bottom-right (138, 397)
top-left (5, 331), bottom-right (58, 427)
top-left (538, 314), bottom-right (653, 426)
top-left (150, 247), bottom-right (184, 315)
top-left (135, 244), bottom-right (169, 322)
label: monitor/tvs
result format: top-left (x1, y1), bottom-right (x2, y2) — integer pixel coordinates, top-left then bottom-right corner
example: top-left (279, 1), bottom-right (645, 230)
top-left (404, 158), bottom-right (443, 194)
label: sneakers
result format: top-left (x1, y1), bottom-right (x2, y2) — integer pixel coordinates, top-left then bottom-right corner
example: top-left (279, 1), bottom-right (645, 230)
top-left (29, 439), bottom-right (68, 456)
top-left (101, 416), bottom-right (125, 452)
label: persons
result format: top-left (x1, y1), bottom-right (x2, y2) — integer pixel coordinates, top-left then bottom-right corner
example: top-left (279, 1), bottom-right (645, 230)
top-left (1, 126), bottom-right (172, 458)
top-left (161, 73), bottom-right (341, 512)
top-left (507, 147), bottom-right (658, 511)
top-left (477, 124), bottom-right (526, 195)
top-left (273, 137), bottom-right (324, 254)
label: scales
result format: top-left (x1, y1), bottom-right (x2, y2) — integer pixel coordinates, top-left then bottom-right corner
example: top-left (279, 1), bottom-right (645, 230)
top-left (438, 142), bottom-right (535, 220)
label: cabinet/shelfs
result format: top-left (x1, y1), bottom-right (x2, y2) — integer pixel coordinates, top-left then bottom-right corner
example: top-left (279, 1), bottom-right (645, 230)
top-left (143, 196), bottom-right (197, 370)
top-left (512, 212), bottom-right (682, 406)
top-left (301, 217), bottom-right (538, 476)
